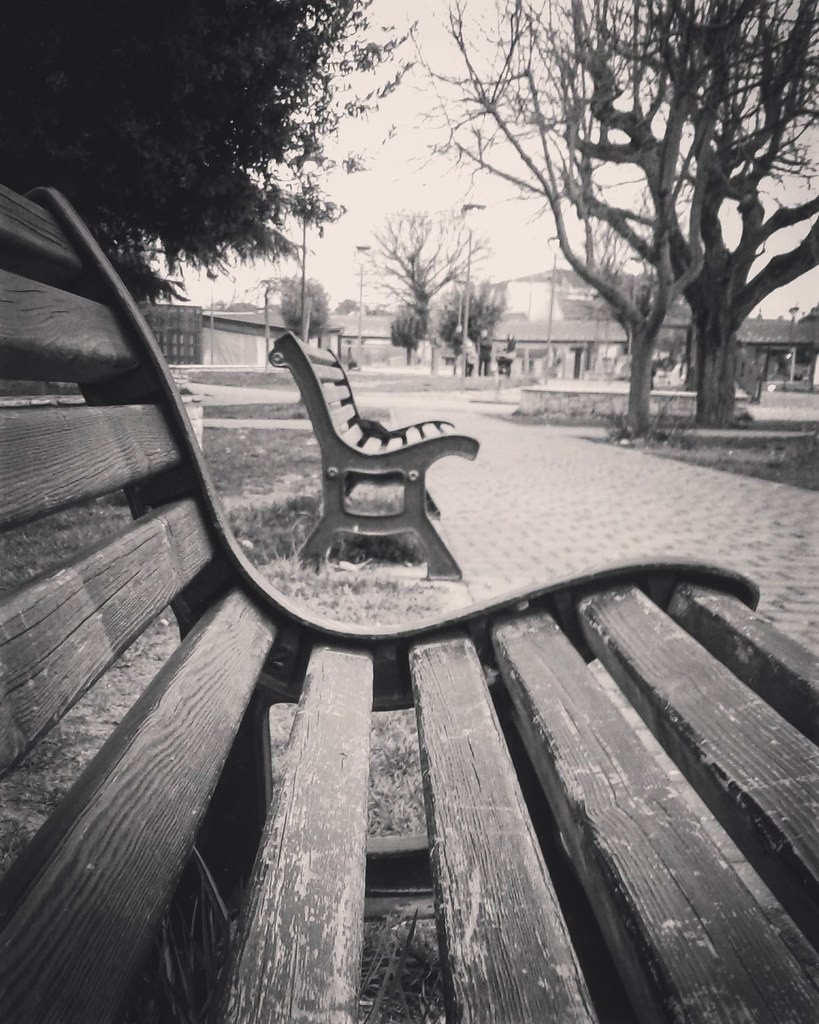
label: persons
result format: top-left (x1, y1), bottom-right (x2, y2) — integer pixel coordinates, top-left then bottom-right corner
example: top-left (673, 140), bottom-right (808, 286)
top-left (475, 328), bottom-right (492, 376)
top-left (498, 332), bottom-right (516, 375)
top-left (451, 324), bottom-right (476, 376)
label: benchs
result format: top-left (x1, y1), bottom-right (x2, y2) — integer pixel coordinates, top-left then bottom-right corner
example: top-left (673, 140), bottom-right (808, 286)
top-left (0, 181), bottom-right (819, 1024)
top-left (266, 330), bottom-right (481, 585)
